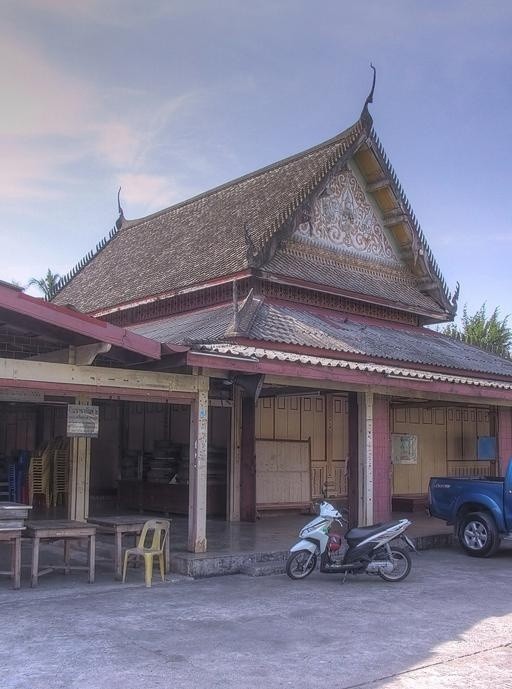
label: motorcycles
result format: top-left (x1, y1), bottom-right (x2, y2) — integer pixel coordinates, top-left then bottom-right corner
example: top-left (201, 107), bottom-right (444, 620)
top-left (285, 496), bottom-right (422, 583)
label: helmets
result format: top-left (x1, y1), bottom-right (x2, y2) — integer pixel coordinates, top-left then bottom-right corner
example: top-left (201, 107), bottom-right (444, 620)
top-left (328, 532), bottom-right (341, 551)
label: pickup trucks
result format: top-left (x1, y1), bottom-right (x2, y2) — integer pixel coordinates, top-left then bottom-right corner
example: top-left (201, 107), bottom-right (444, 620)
top-left (426, 458), bottom-right (512, 557)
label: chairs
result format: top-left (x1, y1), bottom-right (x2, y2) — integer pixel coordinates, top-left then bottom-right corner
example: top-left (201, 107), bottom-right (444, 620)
top-left (8, 435), bottom-right (70, 508)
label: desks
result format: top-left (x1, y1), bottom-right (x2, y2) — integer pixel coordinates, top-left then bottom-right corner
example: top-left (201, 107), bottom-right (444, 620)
top-left (0, 501), bottom-right (174, 590)
